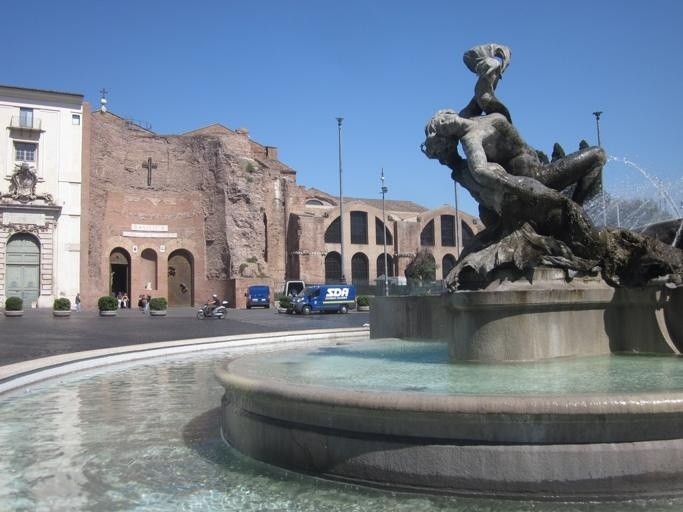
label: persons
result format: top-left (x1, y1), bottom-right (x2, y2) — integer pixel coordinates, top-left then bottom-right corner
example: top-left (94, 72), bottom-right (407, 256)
top-left (112, 289), bottom-right (129, 310)
top-left (423, 107), bottom-right (606, 290)
top-left (208, 294), bottom-right (223, 316)
top-left (137, 294), bottom-right (151, 316)
top-left (76, 293), bottom-right (82, 314)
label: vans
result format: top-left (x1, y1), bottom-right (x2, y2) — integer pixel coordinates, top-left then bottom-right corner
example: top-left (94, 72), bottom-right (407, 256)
top-left (289, 284), bottom-right (357, 315)
top-left (283, 281), bottom-right (305, 302)
top-left (244, 285), bottom-right (269, 309)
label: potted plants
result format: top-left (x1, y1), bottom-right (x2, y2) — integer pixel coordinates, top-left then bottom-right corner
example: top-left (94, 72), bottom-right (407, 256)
top-left (277, 293), bottom-right (292, 313)
top-left (53, 298), bottom-right (71, 317)
top-left (4, 296), bottom-right (25, 317)
top-left (97, 294), bottom-right (119, 316)
top-left (356, 295), bottom-right (370, 312)
top-left (149, 297), bottom-right (168, 317)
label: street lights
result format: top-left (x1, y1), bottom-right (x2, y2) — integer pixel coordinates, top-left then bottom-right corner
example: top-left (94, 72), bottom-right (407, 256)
top-left (379, 168), bottom-right (390, 296)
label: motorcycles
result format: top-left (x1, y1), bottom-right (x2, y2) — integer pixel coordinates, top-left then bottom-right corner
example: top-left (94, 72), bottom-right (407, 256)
top-left (197, 300), bottom-right (229, 320)
